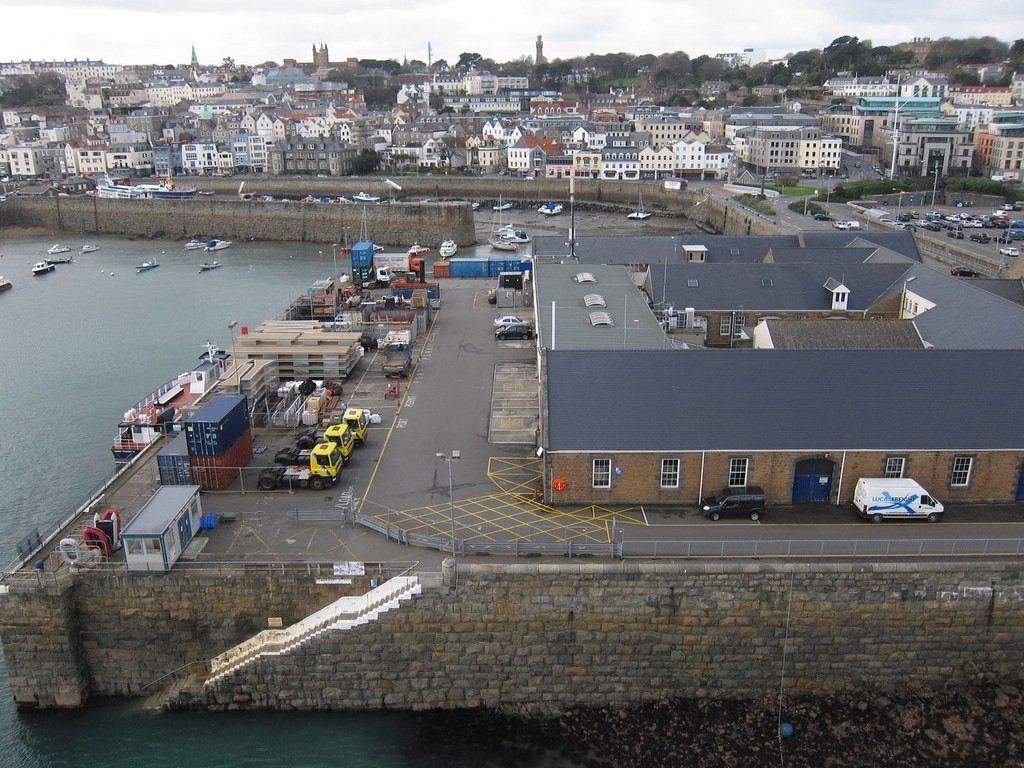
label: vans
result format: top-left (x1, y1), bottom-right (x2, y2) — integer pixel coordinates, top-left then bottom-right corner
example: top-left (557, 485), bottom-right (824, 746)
top-left (851, 477), bottom-right (945, 523)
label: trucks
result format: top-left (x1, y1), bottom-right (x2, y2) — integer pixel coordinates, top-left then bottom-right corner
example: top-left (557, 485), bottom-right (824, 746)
top-left (257, 442), bottom-right (345, 491)
top-left (293, 409), bottom-right (368, 448)
top-left (274, 423), bottom-right (355, 464)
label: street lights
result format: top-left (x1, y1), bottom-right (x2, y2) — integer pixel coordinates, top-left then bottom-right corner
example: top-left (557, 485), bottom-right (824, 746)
top-left (228, 321), bottom-right (242, 395)
top-left (436, 449), bottom-right (462, 558)
top-left (826, 175), bottom-right (833, 206)
top-left (892, 188), bottom-right (903, 222)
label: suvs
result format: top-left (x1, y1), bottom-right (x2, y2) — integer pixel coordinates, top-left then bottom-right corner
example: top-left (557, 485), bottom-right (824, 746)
top-left (698, 486), bottom-right (767, 522)
top-left (494, 323), bottom-right (532, 341)
top-left (832, 220), bottom-right (860, 231)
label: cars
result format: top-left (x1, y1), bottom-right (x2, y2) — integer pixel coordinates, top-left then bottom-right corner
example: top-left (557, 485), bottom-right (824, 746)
top-left (854, 162), bottom-right (861, 168)
top-left (871, 161), bottom-right (884, 176)
top-left (839, 173), bottom-right (850, 180)
top-left (951, 265), bottom-right (980, 279)
top-left (814, 213), bottom-right (833, 222)
top-left (493, 315), bottom-right (531, 329)
top-left (880, 199), bottom-right (1024, 245)
top-left (999, 246), bottom-right (1019, 257)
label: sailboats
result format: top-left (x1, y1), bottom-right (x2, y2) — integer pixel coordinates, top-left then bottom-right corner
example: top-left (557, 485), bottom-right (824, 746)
top-left (359, 206), bottom-right (385, 254)
top-left (626, 187), bottom-right (653, 221)
top-left (490, 194), bottom-right (564, 252)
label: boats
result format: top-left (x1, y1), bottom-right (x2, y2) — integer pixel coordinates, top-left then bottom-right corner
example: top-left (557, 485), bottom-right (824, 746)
top-left (110, 339), bottom-right (234, 465)
top-left (0, 238), bottom-right (232, 295)
top-left (407, 242), bottom-right (431, 258)
top-left (84, 171), bottom-right (481, 211)
top-left (440, 236), bottom-right (459, 261)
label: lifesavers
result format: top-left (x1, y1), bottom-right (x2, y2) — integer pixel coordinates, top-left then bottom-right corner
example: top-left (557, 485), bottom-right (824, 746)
top-left (554, 483), bottom-right (565, 491)
